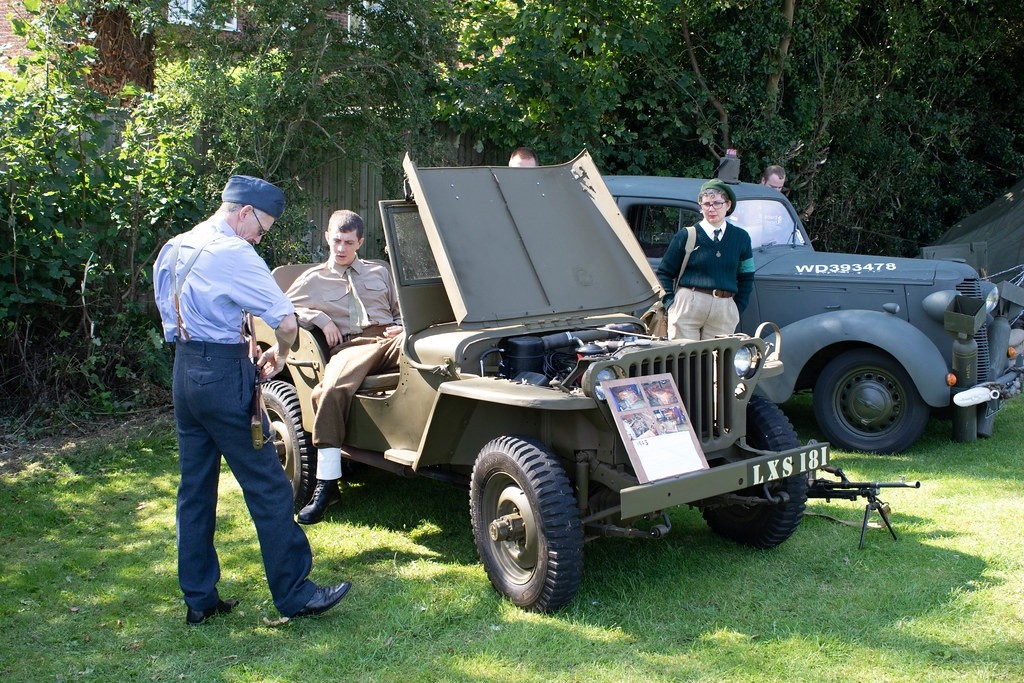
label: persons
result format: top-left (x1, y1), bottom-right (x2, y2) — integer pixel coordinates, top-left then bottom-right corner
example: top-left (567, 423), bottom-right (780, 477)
top-left (507, 146), bottom-right (538, 169)
top-left (150, 175), bottom-right (353, 626)
top-left (286, 209), bottom-right (405, 525)
top-left (746, 166), bottom-right (785, 244)
top-left (656, 178), bottom-right (756, 428)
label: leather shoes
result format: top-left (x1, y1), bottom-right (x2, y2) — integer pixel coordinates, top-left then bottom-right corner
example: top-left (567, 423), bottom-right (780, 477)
top-left (297, 479), bottom-right (342, 524)
top-left (186, 597), bottom-right (239, 625)
top-left (284, 581), bottom-right (352, 619)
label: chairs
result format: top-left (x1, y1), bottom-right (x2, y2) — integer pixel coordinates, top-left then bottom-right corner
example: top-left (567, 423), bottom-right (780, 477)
top-left (272, 259), bottom-right (401, 394)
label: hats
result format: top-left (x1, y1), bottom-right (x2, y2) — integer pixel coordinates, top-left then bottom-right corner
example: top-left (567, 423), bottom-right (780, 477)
top-left (700, 178), bottom-right (737, 217)
top-left (221, 174), bottom-right (286, 220)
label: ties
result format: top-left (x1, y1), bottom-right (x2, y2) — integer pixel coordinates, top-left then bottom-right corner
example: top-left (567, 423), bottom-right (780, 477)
top-left (345, 266), bottom-right (372, 336)
top-left (714, 229), bottom-right (722, 243)
top-left (248, 312), bottom-right (257, 357)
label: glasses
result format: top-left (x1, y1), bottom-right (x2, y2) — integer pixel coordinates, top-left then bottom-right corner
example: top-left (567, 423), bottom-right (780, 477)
top-left (253, 209), bottom-right (267, 237)
top-left (699, 200), bottom-right (728, 211)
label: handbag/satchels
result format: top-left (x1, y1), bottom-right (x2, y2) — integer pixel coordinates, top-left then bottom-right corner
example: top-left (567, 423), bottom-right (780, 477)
top-left (638, 301), bottom-right (668, 339)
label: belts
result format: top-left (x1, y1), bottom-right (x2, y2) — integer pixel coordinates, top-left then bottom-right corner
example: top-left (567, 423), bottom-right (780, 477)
top-left (679, 285), bottom-right (733, 299)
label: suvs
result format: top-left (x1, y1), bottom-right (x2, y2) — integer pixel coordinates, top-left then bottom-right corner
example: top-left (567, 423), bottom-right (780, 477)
top-left (595, 174), bottom-right (1024, 455)
top-left (244, 147), bottom-right (832, 613)
